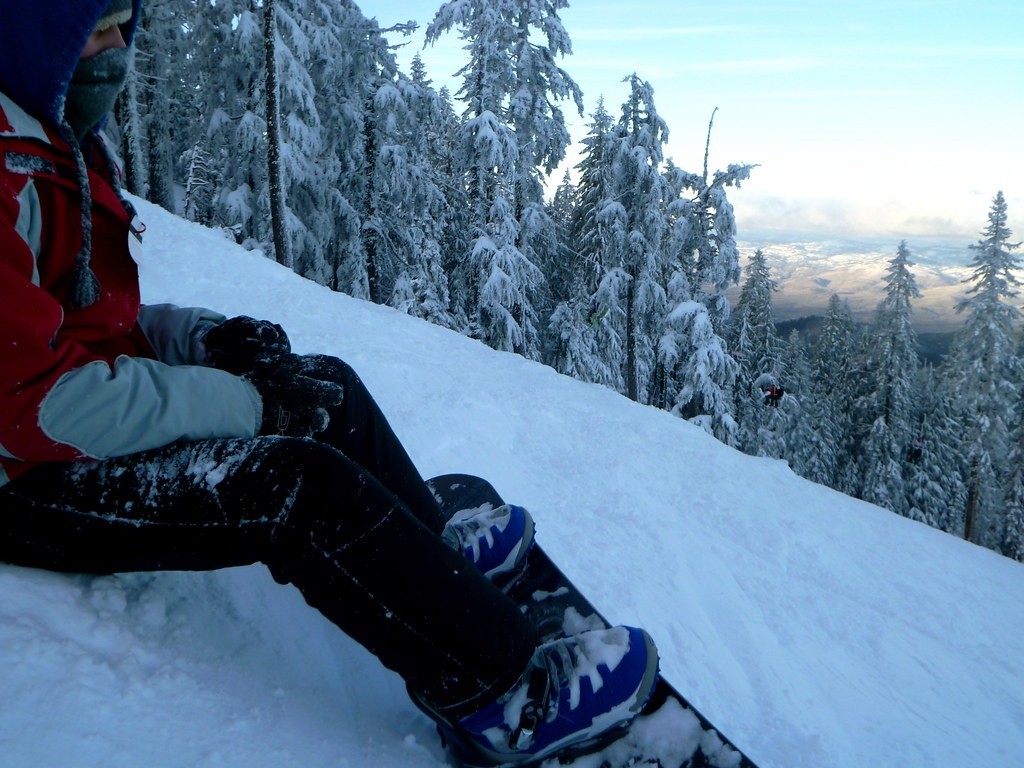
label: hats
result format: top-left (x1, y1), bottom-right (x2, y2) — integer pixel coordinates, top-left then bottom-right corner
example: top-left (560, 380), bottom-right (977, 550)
top-left (0, 0), bottom-right (142, 136)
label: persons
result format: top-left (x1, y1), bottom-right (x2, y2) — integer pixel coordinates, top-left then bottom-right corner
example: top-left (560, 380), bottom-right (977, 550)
top-left (1, 1), bottom-right (659, 768)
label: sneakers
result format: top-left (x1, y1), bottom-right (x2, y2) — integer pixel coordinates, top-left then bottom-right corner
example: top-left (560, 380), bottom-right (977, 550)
top-left (439, 502), bottom-right (537, 594)
top-left (407, 625), bottom-right (661, 768)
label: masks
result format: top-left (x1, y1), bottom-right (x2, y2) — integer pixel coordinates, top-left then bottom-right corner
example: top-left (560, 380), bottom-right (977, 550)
top-left (64, 46), bottom-right (135, 141)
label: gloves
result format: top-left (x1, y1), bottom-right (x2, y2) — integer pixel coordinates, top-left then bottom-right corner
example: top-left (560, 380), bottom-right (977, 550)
top-left (200, 315), bottom-right (291, 376)
top-left (241, 352), bottom-right (344, 438)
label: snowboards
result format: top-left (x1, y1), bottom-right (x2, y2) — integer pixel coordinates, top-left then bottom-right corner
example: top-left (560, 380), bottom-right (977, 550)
top-left (422, 473), bottom-right (759, 768)
